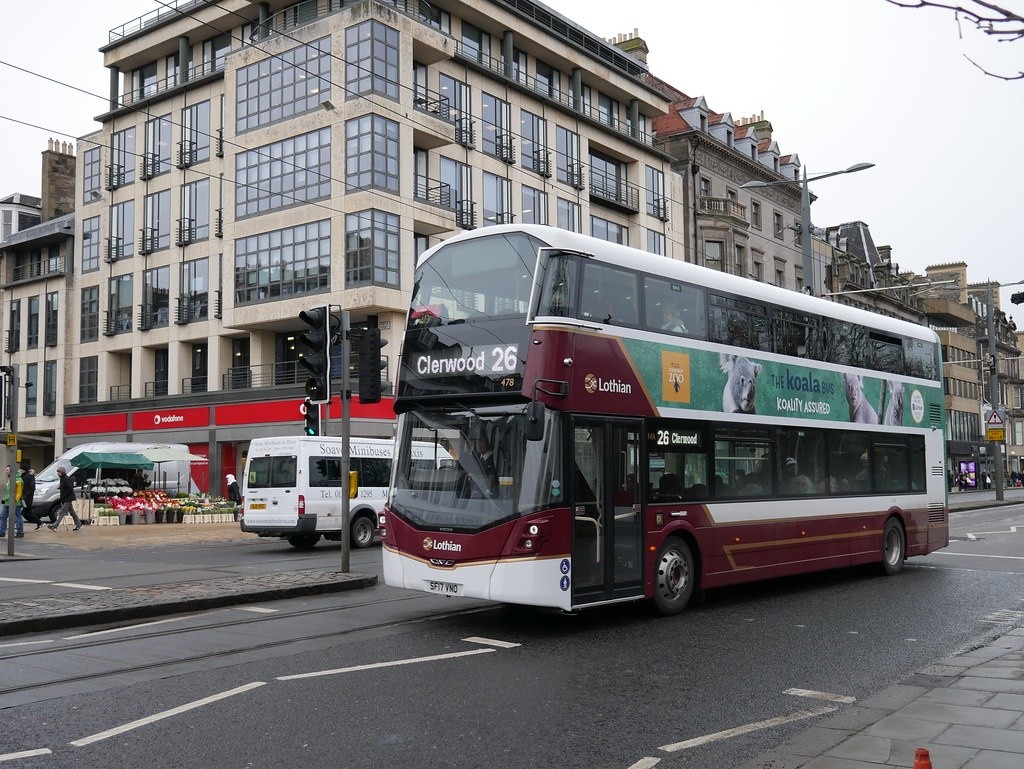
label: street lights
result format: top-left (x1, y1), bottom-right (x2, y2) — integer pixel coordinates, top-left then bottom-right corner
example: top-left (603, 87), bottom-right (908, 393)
top-left (943, 278), bottom-right (1024, 500)
top-left (737, 162), bottom-right (877, 294)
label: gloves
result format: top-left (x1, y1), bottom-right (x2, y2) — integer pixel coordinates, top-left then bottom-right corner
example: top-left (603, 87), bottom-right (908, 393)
top-left (59, 498), bottom-right (63, 502)
top-left (1, 500), bottom-right (5, 504)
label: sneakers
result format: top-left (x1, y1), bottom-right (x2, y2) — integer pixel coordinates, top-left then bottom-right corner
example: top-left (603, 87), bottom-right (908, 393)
top-left (35, 522), bottom-right (45, 530)
top-left (72, 524), bottom-right (83, 531)
top-left (47, 525), bottom-right (57, 532)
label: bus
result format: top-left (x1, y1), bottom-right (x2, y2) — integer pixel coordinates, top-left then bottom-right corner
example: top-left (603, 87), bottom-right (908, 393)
top-left (377, 220), bottom-right (952, 617)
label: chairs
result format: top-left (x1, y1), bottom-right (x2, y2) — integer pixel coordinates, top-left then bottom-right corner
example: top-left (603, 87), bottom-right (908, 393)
top-left (680, 484), bottom-right (708, 503)
top-left (655, 473), bottom-right (685, 501)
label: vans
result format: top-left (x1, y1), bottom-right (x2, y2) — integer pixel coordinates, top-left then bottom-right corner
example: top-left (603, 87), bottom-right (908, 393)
top-left (239, 435), bottom-right (457, 550)
top-left (21, 441), bottom-right (193, 519)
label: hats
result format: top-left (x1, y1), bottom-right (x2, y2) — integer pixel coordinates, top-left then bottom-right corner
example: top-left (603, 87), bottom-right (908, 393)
top-left (57, 467), bottom-right (66, 474)
top-left (785, 457), bottom-right (797, 465)
top-left (20, 463), bottom-right (30, 471)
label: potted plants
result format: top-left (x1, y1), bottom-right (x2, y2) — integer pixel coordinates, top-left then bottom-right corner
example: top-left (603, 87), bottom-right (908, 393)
top-left (98, 510), bottom-right (119, 526)
top-left (176, 492), bottom-right (189, 505)
top-left (194, 492), bottom-right (235, 522)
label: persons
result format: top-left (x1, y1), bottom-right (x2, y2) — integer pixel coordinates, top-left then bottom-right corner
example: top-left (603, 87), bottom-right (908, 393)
top-left (1005, 471), bottom-right (1024, 488)
top-left (947, 469), bottom-right (954, 492)
top-left (958, 471), bottom-right (971, 492)
top-left (0, 465), bottom-right (25, 538)
top-left (455, 430), bottom-right (502, 499)
top-left (47, 465), bottom-right (83, 531)
top-left (660, 303), bottom-right (688, 333)
top-left (982, 470), bottom-right (992, 490)
top-left (715, 453), bottom-right (859, 496)
top-left (225, 473), bottom-right (242, 521)
top-left (131, 469), bottom-right (152, 491)
top-left (18, 463), bottom-right (45, 530)
top-left (834, 343), bottom-right (855, 365)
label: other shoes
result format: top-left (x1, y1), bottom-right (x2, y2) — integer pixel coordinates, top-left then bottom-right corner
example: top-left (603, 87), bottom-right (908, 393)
top-left (0, 532), bottom-right (6, 538)
top-left (14, 533), bottom-right (24, 538)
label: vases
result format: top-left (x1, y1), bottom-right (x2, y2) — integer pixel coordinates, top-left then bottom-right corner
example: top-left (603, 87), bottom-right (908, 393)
top-left (131, 511), bottom-right (139, 525)
top-left (167, 510), bottom-right (175, 523)
top-left (177, 510), bottom-right (184, 523)
top-left (119, 512), bottom-right (127, 525)
top-left (146, 513), bottom-right (154, 524)
top-left (155, 510), bottom-right (163, 524)
top-left (185, 510), bottom-right (193, 514)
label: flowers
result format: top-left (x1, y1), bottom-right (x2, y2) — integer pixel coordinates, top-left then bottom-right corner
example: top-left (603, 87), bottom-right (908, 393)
top-left (86, 477), bottom-right (182, 511)
top-left (184, 506), bottom-right (196, 511)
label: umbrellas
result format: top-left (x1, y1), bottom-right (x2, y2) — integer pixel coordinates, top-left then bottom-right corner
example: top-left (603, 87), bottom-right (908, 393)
top-left (138, 443), bottom-right (209, 489)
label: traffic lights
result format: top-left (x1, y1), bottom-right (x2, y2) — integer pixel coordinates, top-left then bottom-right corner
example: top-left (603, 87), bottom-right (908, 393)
top-left (298, 303), bottom-right (331, 405)
top-left (358, 327), bottom-right (389, 403)
top-left (302, 397), bottom-right (322, 436)
